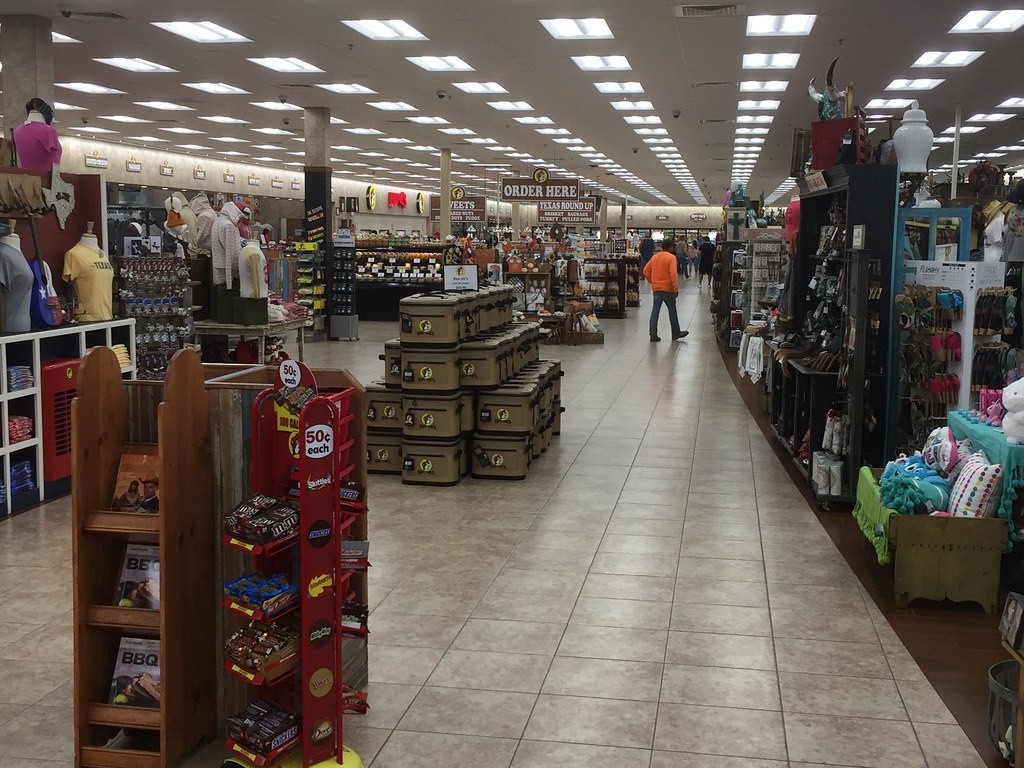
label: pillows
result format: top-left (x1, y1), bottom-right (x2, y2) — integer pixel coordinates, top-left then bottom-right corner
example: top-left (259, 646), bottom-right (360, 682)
top-left (940, 439), bottom-right (1003, 519)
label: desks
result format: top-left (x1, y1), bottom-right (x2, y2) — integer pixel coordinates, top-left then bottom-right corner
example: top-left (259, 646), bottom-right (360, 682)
top-left (192, 318), bottom-right (312, 366)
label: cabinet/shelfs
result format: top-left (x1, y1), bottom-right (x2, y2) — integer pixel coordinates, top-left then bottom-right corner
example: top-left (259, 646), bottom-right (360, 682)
top-left (353, 238), bottom-right (627, 321)
top-left (0, 318), bottom-right (367, 768)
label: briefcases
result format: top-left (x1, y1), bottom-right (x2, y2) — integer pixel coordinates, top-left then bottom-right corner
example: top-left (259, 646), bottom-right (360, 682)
top-left (363, 282), bottom-right (566, 486)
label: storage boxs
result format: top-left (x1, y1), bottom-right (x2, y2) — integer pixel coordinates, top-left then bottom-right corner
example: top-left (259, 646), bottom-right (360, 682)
top-left (864, 466), bottom-right (1009, 614)
top-left (503, 272), bottom-right (551, 313)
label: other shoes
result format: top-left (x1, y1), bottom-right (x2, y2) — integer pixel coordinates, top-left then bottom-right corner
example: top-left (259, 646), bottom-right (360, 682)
top-left (672, 331), bottom-right (689, 340)
top-left (650, 335), bottom-right (661, 342)
top-left (697, 283), bottom-right (702, 287)
top-left (707, 284), bottom-right (710, 287)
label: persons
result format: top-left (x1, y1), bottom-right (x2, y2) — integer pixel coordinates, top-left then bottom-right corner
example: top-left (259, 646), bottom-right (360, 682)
top-left (139, 472), bottom-right (160, 514)
top-left (639, 233), bottom-right (717, 288)
top-left (0, 218), bottom-right (34, 333)
top-left (13, 97), bottom-right (62, 173)
top-left (62, 221), bottom-right (115, 320)
top-left (165, 189), bottom-right (269, 299)
top-left (643, 239), bottom-right (689, 342)
top-left (786, 195), bottom-right (801, 244)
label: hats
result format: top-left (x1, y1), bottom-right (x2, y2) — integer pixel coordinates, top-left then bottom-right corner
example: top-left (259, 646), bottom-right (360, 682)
top-left (166, 210), bottom-right (186, 227)
top-left (662, 239), bottom-right (675, 248)
top-left (1007, 179), bottom-right (1024, 204)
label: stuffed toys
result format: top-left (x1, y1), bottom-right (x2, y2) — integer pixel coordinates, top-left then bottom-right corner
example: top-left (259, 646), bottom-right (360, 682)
top-left (986, 399), bottom-right (1001, 427)
top-left (879, 454), bottom-right (950, 513)
top-left (1001, 376), bottom-right (1024, 445)
